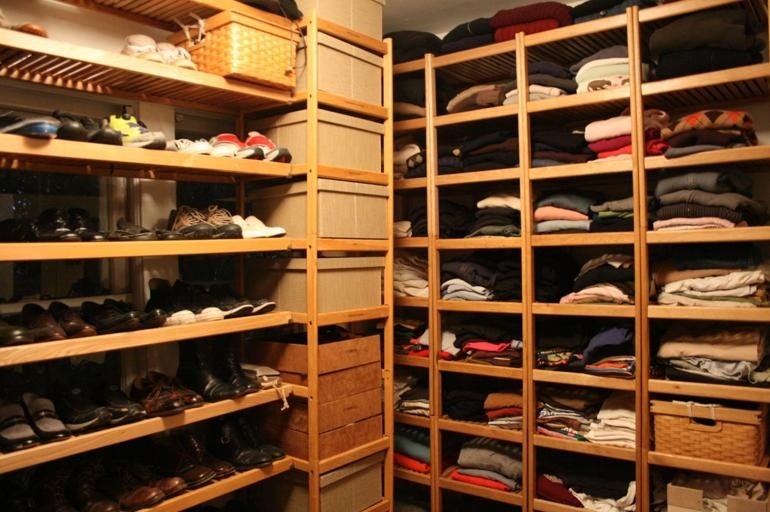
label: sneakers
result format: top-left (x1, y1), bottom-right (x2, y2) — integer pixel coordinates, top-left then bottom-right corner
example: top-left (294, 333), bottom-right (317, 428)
top-left (1, 110), bottom-right (292, 164)
top-left (122, 35), bottom-right (196, 70)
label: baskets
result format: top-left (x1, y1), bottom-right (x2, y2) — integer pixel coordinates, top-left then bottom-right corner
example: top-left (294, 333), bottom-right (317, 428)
top-left (648, 400), bottom-right (767, 466)
top-left (164, 10), bottom-right (297, 90)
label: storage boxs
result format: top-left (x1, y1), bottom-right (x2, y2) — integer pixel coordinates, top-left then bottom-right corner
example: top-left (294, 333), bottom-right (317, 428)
top-left (249, 0), bottom-right (384, 512)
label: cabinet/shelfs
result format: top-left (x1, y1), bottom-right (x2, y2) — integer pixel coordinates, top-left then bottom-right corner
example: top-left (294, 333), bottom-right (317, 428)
top-left (0, 0), bottom-right (398, 511)
top-left (396, 1), bottom-right (770, 512)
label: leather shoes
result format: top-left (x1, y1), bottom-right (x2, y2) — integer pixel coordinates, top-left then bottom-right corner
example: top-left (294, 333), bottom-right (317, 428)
top-left (1, 356), bottom-right (259, 449)
top-left (0, 277), bottom-right (276, 344)
top-left (0, 205), bottom-right (286, 243)
top-left (1, 418), bottom-right (286, 511)
